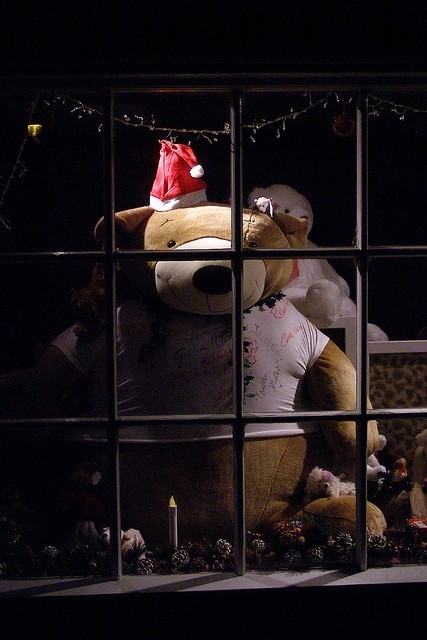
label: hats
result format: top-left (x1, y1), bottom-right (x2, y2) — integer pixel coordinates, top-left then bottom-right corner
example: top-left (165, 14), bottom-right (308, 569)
top-left (150, 142), bottom-right (206, 211)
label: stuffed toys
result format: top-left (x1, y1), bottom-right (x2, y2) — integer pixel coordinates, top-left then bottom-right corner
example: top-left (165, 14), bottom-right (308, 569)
top-left (303, 466), bottom-right (355, 503)
top-left (326, 434), bottom-right (389, 503)
top-left (253, 197), bottom-right (269, 213)
top-left (99, 527), bottom-right (145, 560)
top-left (37, 140), bottom-right (385, 549)
top-left (248, 185), bottom-right (388, 340)
top-left (390, 458), bottom-right (407, 482)
top-left (410, 429), bottom-right (426, 518)
